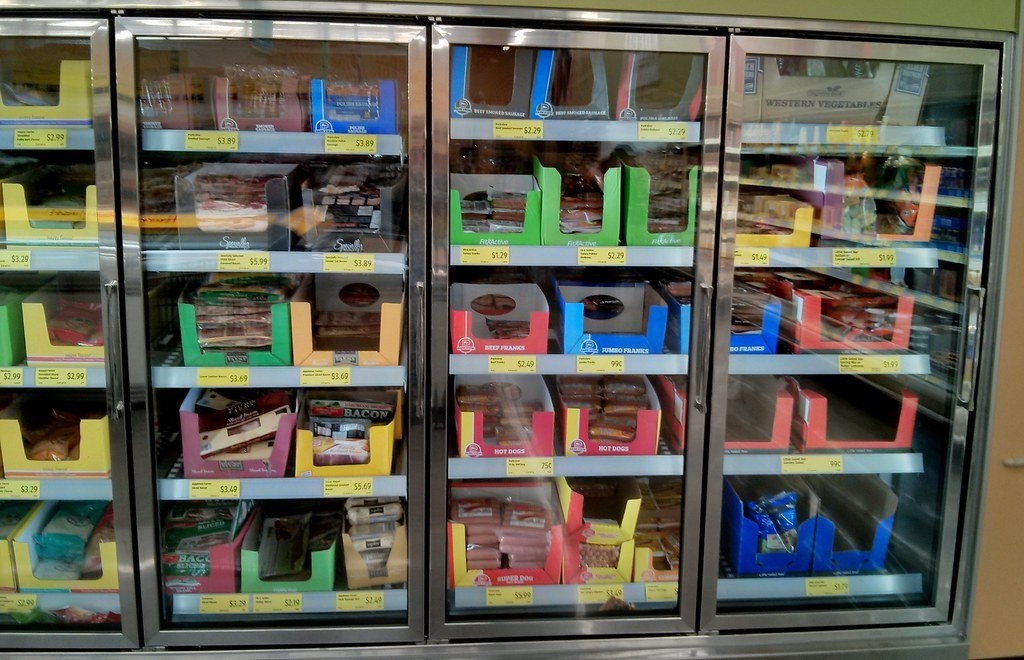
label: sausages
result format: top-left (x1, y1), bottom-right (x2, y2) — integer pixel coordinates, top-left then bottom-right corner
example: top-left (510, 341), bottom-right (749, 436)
top-left (450, 497), bottom-right (554, 570)
